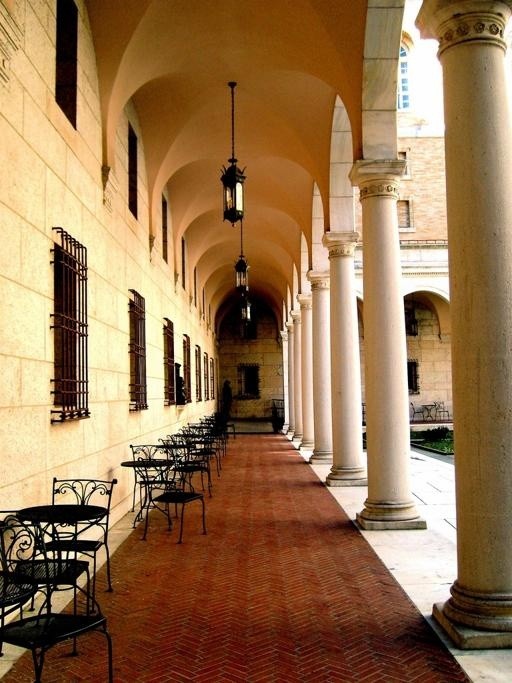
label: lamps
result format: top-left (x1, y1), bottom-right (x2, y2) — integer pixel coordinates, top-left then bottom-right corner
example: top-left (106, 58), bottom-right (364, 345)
top-left (217, 77), bottom-right (253, 329)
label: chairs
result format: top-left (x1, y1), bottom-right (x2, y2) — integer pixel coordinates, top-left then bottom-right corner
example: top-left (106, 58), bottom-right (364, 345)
top-left (410, 400), bottom-right (451, 424)
top-left (122, 403), bottom-right (237, 542)
top-left (1, 479), bottom-right (123, 681)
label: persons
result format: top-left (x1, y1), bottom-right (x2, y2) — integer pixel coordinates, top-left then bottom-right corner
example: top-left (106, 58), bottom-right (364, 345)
top-left (223, 380), bottom-right (237, 418)
